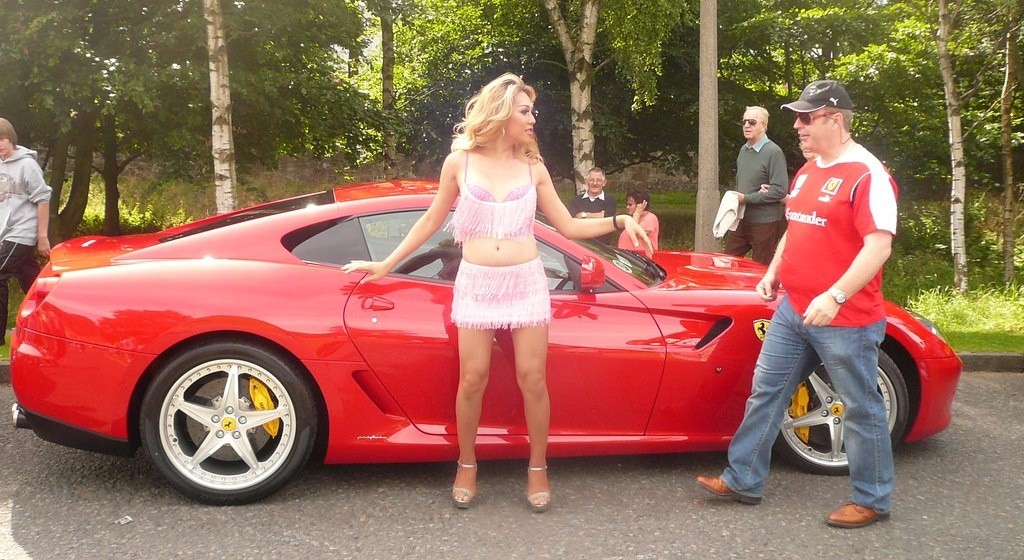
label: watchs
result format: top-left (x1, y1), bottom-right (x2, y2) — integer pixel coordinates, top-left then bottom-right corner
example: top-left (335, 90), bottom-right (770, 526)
top-left (829, 286), bottom-right (848, 306)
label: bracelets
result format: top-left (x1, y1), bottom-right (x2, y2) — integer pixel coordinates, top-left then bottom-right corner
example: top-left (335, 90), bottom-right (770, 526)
top-left (613, 214), bottom-right (622, 230)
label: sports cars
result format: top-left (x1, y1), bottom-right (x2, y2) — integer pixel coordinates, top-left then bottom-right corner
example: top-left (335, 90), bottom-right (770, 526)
top-left (7, 181), bottom-right (964, 504)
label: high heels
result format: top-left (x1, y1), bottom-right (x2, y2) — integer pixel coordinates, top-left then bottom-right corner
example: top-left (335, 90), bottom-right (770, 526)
top-left (452, 459), bottom-right (478, 509)
top-left (526, 465), bottom-right (551, 513)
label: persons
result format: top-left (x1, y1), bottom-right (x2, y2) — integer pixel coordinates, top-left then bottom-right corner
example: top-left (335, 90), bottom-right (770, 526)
top-left (0, 117), bottom-right (55, 347)
top-left (339, 72), bottom-right (655, 513)
top-left (618, 189), bottom-right (659, 251)
top-left (569, 167), bottom-right (616, 247)
top-left (680, 81), bottom-right (901, 529)
top-left (722, 106), bottom-right (788, 268)
top-left (758, 180), bottom-right (793, 203)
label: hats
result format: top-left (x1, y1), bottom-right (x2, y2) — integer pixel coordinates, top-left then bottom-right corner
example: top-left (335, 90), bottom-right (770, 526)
top-left (780, 79), bottom-right (854, 113)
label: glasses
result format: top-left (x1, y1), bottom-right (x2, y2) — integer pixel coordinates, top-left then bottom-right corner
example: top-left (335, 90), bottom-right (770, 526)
top-left (742, 119), bottom-right (763, 126)
top-left (793, 110), bottom-right (838, 126)
top-left (626, 202), bottom-right (639, 207)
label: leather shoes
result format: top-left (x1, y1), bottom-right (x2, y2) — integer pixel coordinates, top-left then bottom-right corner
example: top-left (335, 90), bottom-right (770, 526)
top-left (828, 501), bottom-right (890, 529)
top-left (695, 474), bottom-right (763, 506)
